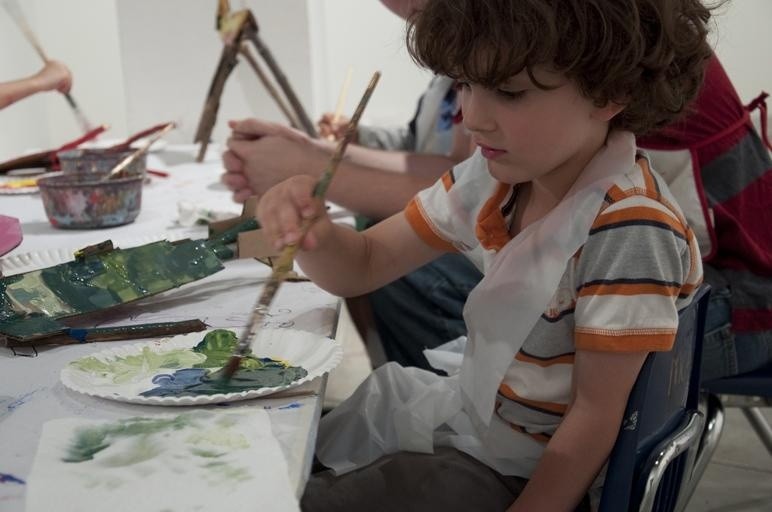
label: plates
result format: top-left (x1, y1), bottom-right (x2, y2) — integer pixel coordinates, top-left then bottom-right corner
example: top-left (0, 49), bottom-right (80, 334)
top-left (58, 328), bottom-right (343, 407)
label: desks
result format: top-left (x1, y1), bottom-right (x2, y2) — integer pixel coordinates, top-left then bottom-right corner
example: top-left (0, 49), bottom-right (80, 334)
top-left (0, 140), bottom-right (356, 511)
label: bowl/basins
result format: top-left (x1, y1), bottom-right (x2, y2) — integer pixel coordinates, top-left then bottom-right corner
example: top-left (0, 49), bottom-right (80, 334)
top-left (38, 173), bottom-right (142, 231)
top-left (57, 149), bottom-right (146, 181)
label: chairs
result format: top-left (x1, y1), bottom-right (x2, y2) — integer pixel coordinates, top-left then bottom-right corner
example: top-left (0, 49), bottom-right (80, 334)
top-left (714, 392), bottom-right (772, 457)
top-left (590, 282), bottom-right (729, 511)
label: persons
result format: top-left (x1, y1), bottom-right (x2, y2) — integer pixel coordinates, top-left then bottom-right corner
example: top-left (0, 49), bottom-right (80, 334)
top-left (256, 1), bottom-right (709, 512)
top-left (0, 60), bottom-right (72, 110)
top-left (220, 1), bottom-right (772, 384)
top-left (316, 72), bottom-right (461, 233)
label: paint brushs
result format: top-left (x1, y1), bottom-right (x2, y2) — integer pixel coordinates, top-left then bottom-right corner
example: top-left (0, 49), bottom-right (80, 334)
top-left (326, 69), bottom-right (353, 145)
top-left (218, 69), bottom-right (384, 385)
top-left (113, 123), bottom-right (167, 150)
top-left (43, 126), bottom-right (106, 161)
top-left (16, 17), bottom-right (93, 135)
top-left (98, 124), bottom-right (176, 181)
